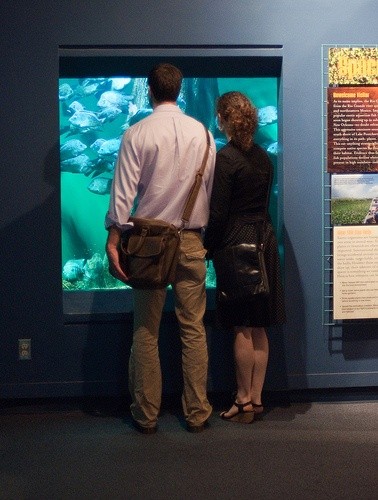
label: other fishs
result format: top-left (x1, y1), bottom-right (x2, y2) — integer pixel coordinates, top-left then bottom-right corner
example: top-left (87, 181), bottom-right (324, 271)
top-left (60, 77), bottom-right (154, 196)
top-left (259, 105), bottom-right (277, 155)
top-left (62, 250), bottom-right (110, 283)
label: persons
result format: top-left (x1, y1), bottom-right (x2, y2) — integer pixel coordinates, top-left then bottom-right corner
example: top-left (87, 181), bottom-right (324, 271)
top-left (202, 92), bottom-right (285, 424)
top-left (104, 62), bottom-right (217, 434)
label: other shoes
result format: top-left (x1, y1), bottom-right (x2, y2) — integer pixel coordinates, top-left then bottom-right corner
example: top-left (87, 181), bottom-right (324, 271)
top-left (186, 421), bottom-right (208, 433)
top-left (131, 419), bottom-right (156, 433)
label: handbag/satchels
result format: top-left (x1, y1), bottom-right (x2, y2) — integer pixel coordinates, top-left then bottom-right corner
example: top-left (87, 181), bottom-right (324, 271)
top-left (107, 217), bottom-right (182, 291)
top-left (213, 242), bottom-right (271, 305)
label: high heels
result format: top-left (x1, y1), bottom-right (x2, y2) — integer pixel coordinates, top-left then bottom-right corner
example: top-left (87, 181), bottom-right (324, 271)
top-left (219, 401), bottom-right (265, 425)
